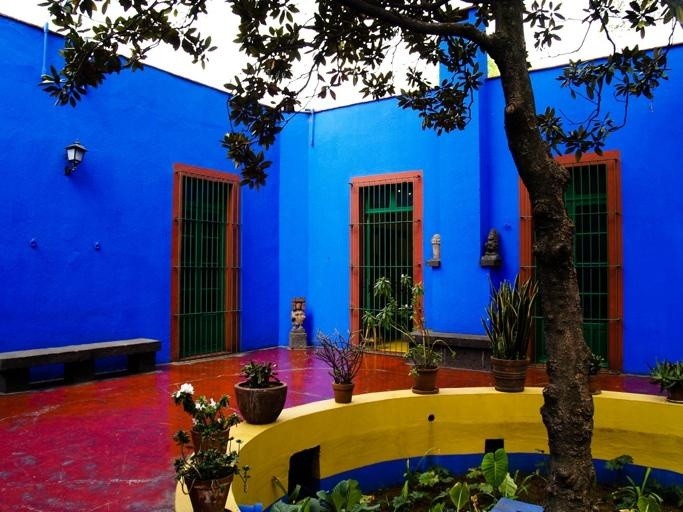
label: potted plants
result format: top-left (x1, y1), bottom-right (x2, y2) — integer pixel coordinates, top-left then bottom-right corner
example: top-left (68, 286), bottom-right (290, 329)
top-left (234, 359), bottom-right (288, 424)
top-left (480, 274), bottom-right (540, 392)
top-left (363, 274), bottom-right (456, 394)
top-left (588, 353), bottom-right (605, 394)
top-left (648, 356), bottom-right (683, 403)
top-left (172, 429), bottom-right (249, 512)
top-left (315, 327), bottom-right (368, 403)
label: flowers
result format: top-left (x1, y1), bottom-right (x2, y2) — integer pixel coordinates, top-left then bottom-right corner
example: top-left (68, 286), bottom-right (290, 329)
top-left (170, 383), bottom-right (242, 430)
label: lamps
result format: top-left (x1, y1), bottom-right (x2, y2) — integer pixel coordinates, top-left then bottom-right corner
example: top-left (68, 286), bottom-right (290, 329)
top-left (65, 137), bottom-right (88, 175)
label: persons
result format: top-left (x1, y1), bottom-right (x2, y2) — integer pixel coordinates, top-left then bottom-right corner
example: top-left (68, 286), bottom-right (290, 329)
top-left (430, 234), bottom-right (439, 260)
top-left (484, 226), bottom-right (500, 258)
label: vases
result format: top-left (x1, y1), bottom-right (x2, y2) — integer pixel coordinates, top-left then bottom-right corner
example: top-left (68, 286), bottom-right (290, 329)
top-left (191, 430), bottom-right (229, 456)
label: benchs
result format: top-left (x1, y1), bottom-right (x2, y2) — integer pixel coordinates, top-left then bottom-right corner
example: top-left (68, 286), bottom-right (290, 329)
top-left (0, 337), bottom-right (160, 393)
top-left (405, 330), bottom-right (491, 370)
